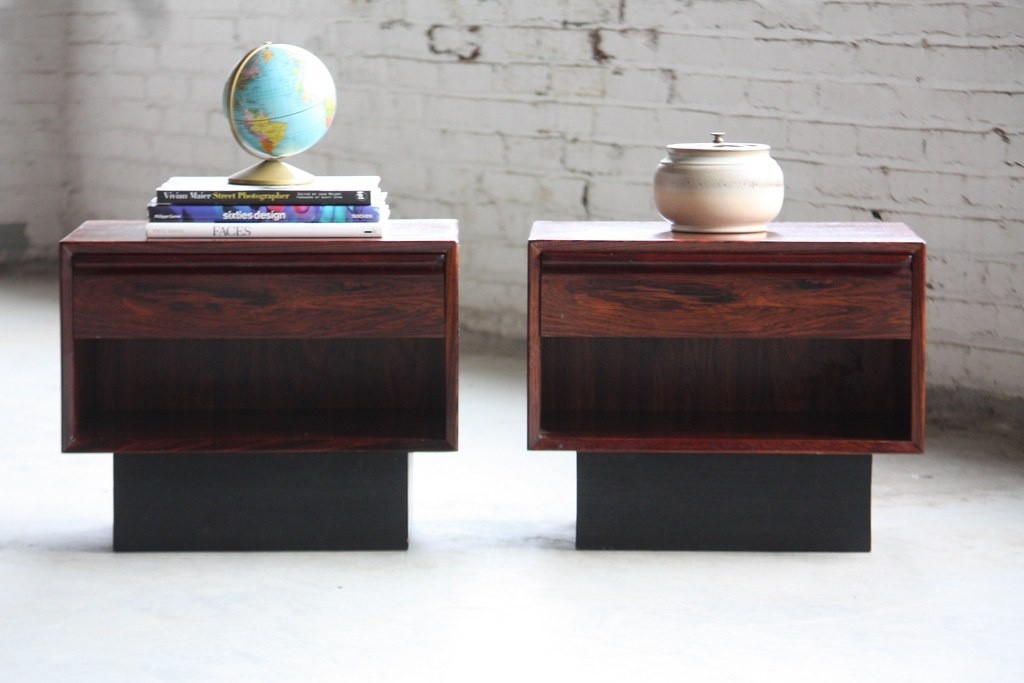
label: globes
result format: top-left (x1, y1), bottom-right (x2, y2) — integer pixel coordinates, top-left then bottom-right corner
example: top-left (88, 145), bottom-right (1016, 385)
top-left (224, 42), bottom-right (343, 187)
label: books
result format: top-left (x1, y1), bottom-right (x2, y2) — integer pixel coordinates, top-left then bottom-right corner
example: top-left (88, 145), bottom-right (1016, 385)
top-left (146, 177), bottom-right (390, 238)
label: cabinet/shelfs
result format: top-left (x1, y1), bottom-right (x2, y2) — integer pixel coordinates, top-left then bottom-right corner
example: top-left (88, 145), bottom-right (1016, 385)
top-left (524, 221), bottom-right (930, 451)
top-left (55, 218), bottom-right (460, 454)
